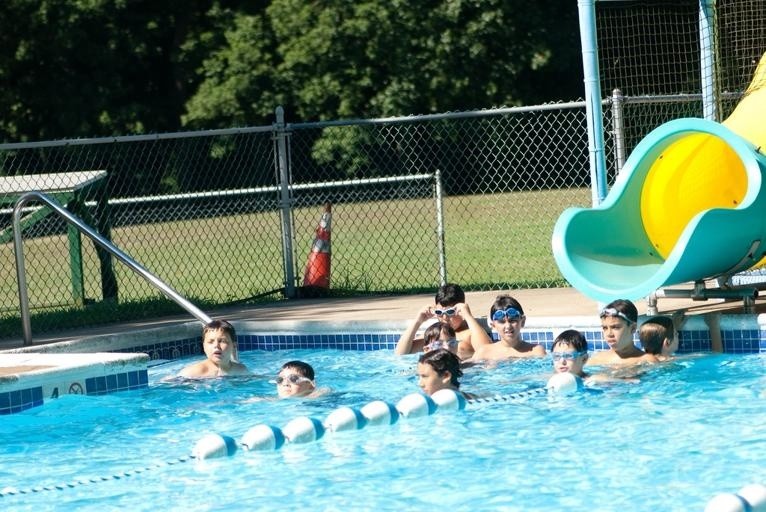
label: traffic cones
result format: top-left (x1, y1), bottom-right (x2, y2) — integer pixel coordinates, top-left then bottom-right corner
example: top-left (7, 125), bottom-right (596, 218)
top-left (282, 201), bottom-right (338, 299)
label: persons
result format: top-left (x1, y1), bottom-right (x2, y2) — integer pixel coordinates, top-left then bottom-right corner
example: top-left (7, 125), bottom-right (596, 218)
top-left (240, 361), bottom-right (334, 404)
top-left (161, 320), bottom-right (248, 384)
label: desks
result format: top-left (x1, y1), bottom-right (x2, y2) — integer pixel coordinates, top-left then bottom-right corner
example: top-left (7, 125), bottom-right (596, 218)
top-left (1, 169), bottom-right (117, 311)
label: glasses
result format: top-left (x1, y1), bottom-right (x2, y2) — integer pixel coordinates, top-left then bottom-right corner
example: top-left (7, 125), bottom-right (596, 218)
top-left (435, 309), bottom-right (455, 316)
top-left (599, 309), bottom-right (633, 324)
top-left (274, 374), bottom-right (298, 383)
top-left (492, 308), bottom-right (521, 322)
top-left (549, 352), bottom-right (587, 359)
top-left (430, 340), bottom-right (458, 350)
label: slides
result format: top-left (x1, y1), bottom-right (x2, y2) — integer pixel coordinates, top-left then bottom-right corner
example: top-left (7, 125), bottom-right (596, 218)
top-left (551, 52), bottom-right (766, 304)
